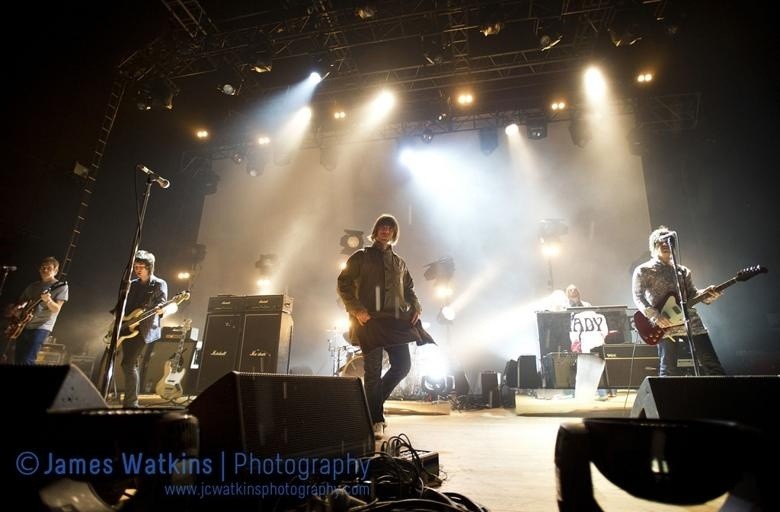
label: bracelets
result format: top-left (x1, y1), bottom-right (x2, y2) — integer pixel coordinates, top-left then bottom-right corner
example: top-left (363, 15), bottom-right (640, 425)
top-left (46, 299), bottom-right (52, 305)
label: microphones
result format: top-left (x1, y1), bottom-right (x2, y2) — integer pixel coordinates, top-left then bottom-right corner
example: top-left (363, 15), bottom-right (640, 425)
top-left (136, 159), bottom-right (171, 190)
top-left (3, 265), bottom-right (17, 272)
top-left (658, 231), bottom-right (678, 242)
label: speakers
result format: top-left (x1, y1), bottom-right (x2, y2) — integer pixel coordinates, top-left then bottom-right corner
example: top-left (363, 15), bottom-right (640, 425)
top-left (626, 374), bottom-right (779, 419)
top-left (193, 294), bottom-right (243, 394)
top-left (236, 294), bottom-right (296, 374)
top-left (516, 355), bottom-right (539, 388)
top-left (589, 342), bottom-right (708, 390)
top-left (0, 361), bottom-right (110, 413)
top-left (454, 370), bottom-right (471, 395)
top-left (186, 370), bottom-right (376, 471)
top-left (136, 326), bottom-right (200, 395)
top-left (504, 360), bottom-right (517, 387)
top-left (480, 371), bottom-right (502, 402)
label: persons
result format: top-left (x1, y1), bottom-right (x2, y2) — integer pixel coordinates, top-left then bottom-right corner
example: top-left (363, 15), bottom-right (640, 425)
top-left (565, 285), bottom-right (593, 308)
top-left (120, 250), bottom-right (168, 409)
top-left (13, 258), bottom-right (71, 364)
top-left (336, 211), bottom-right (441, 441)
top-left (633, 225), bottom-right (732, 375)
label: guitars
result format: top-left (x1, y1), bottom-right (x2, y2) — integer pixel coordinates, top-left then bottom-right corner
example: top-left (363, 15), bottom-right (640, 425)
top-left (4, 279), bottom-right (68, 339)
top-left (106, 290), bottom-right (190, 350)
top-left (155, 318), bottom-right (192, 400)
top-left (634, 265), bottom-right (768, 346)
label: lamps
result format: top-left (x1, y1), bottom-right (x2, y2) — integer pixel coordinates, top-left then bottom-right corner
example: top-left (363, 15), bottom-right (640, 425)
top-left (134, 0), bottom-right (642, 178)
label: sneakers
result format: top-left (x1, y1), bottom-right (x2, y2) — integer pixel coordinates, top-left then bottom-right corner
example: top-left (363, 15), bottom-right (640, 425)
top-left (373, 421), bottom-right (385, 439)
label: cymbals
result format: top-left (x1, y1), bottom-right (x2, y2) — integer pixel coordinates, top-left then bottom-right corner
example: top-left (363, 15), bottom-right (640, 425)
top-left (344, 331), bottom-right (350, 344)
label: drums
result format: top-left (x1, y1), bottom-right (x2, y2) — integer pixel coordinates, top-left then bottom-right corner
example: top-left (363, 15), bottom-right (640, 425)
top-left (343, 356), bottom-right (365, 393)
